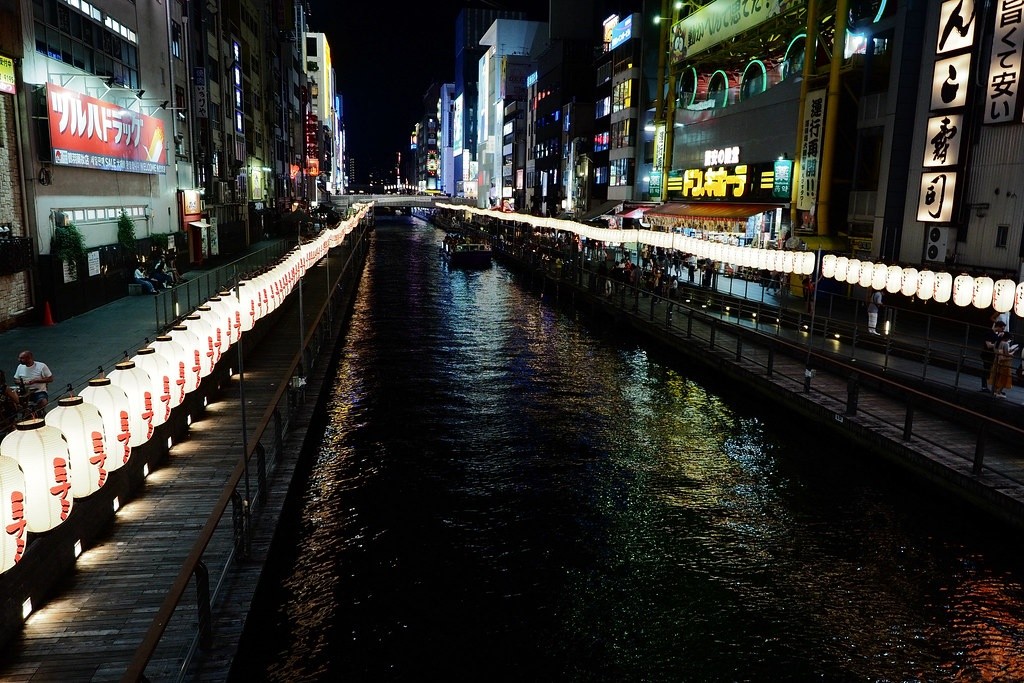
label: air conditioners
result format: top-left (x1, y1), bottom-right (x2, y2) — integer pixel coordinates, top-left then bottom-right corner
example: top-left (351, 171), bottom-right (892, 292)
top-left (926, 225), bottom-right (957, 262)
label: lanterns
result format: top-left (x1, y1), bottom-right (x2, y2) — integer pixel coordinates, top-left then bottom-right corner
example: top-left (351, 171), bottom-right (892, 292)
top-left (2, 202), bottom-right (1024, 579)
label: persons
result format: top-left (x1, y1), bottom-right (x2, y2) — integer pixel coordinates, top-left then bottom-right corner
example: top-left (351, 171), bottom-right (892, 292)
top-left (0, 370), bottom-right (19, 423)
top-left (149, 184), bottom-right (768, 319)
top-left (134, 264), bottom-right (158, 294)
top-left (13, 350), bottom-right (54, 409)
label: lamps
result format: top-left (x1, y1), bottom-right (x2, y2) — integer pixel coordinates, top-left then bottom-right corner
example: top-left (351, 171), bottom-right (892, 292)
top-left (85, 83), bottom-right (145, 101)
top-left (119, 96), bottom-right (169, 110)
top-left (140, 106), bottom-right (187, 119)
top-left (48, 72), bottom-right (117, 90)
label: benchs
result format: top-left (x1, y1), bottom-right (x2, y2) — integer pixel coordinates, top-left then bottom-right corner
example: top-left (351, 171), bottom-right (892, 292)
top-left (127, 284), bottom-right (143, 298)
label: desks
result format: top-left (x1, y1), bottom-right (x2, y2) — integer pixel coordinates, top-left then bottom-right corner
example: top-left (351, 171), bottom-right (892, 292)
top-left (11, 387), bottom-right (39, 419)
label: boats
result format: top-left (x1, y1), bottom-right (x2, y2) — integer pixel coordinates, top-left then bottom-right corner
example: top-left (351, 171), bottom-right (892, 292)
top-left (440, 239), bottom-right (492, 268)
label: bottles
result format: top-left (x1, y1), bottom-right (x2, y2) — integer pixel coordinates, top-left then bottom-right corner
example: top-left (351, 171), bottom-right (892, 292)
top-left (18, 378), bottom-right (25, 394)
